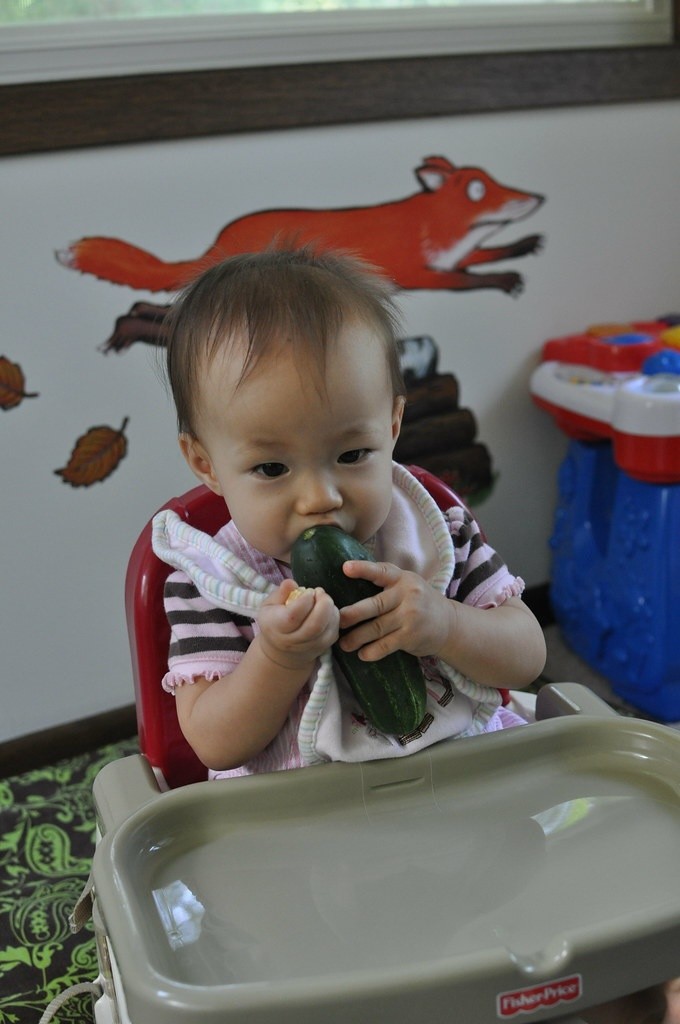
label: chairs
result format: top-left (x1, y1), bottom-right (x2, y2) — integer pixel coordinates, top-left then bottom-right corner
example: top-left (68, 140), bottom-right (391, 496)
top-left (117, 459), bottom-right (538, 786)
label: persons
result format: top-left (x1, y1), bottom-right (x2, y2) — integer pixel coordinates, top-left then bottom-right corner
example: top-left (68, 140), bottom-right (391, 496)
top-left (158, 242), bottom-right (547, 779)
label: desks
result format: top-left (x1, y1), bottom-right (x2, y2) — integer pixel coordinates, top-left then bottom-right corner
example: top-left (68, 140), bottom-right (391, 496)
top-left (93, 684), bottom-right (680, 1024)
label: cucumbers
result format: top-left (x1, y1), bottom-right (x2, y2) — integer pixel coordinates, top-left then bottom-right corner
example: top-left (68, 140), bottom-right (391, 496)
top-left (291, 524), bottom-right (428, 738)
